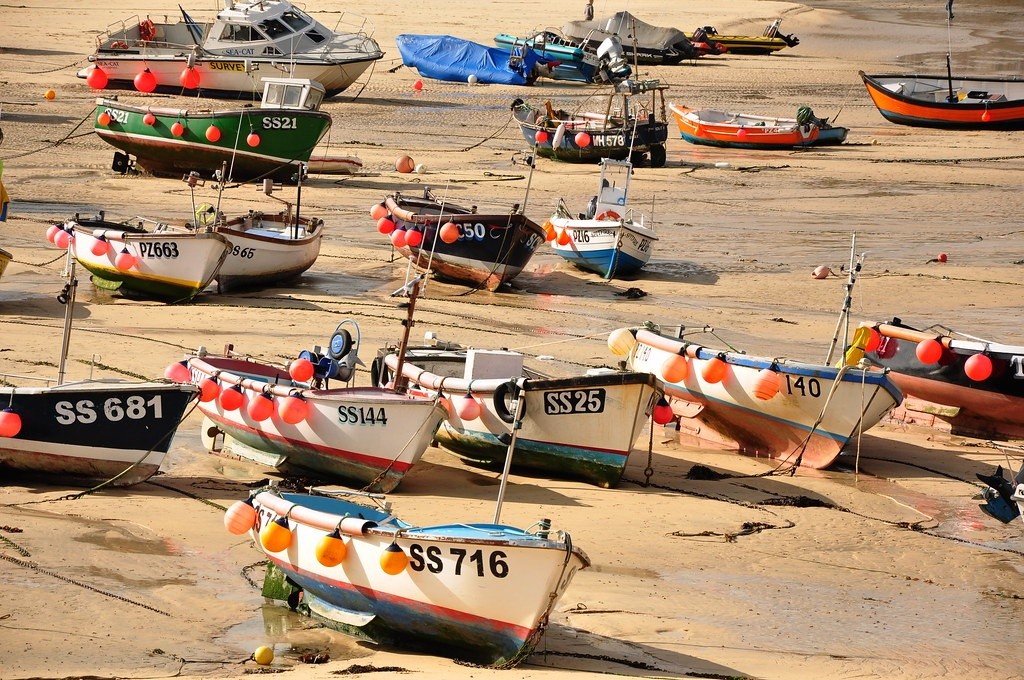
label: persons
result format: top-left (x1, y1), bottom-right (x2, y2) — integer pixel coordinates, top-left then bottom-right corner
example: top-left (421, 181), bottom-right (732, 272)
top-left (582, 0), bottom-right (596, 21)
top-left (586, 195), bottom-right (597, 219)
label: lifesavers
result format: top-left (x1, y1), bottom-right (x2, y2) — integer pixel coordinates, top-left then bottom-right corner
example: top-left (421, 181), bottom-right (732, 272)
top-left (370, 357), bottom-right (389, 388)
top-left (493, 382), bottom-right (526, 424)
top-left (139, 19), bottom-right (155, 41)
top-left (596, 210), bottom-right (622, 222)
top-left (111, 41), bottom-right (128, 49)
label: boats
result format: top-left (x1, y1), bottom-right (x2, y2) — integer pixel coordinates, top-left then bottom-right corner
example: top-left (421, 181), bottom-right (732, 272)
top-left (681, 17), bottom-right (802, 56)
top-left (667, 100), bottom-right (823, 151)
top-left (376, 328), bottom-right (664, 497)
top-left (808, 120), bottom-right (853, 147)
top-left (606, 233), bottom-right (903, 471)
top-left (538, 107), bottom-right (663, 282)
top-left (41, 156), bottom-right (234, 302)
top-left (842, 312), bottom-right (1024, 443)
top-left (556, 7), bottom-right (698, 67)
top-left (857, 47), bottom-right (1024, 132)
top-left (0, 262), bottom-right (205, 489)
top-left (510, 67), bottom-right (669, 168)
top-left (73, 0), bottom-right (387, 100)
top-left (205, 158), bottom-right (326, 294)
top-left (89, 33), bottom-right (336, 183)
top-left (164, 268), bottom-right (457, 495)
top-left (369, 140), bottom-right (549, 294)
top-left (395, 33), bottom-right (540, 85)
top-left (493, 19), bottom-right (635, 86)
top-left (306, 153), bottom-right (367, 178)
top-left (220, 382), bottom-right (590, 668)
